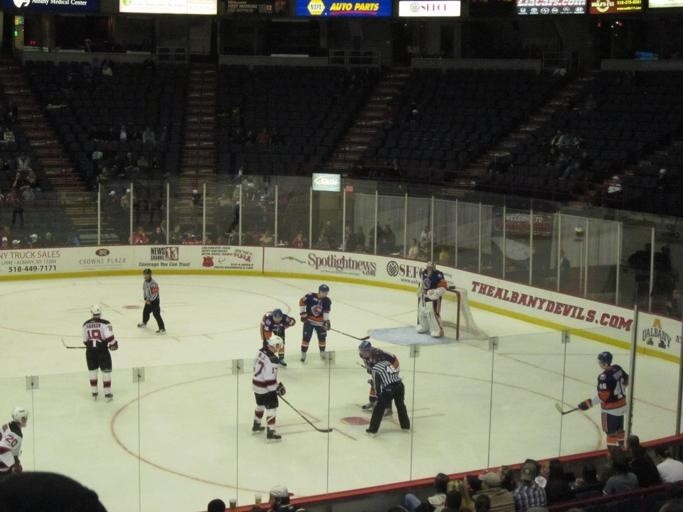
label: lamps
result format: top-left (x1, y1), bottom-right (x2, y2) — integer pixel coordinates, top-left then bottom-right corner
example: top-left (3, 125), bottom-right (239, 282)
top-left (388, 478), bottom-right (683, 512)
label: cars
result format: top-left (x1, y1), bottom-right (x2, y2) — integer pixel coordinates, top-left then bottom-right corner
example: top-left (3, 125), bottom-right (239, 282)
top-left (254, 495), bottom-right (261, 507)
top-left (229, 500), bottom-right (236, 509)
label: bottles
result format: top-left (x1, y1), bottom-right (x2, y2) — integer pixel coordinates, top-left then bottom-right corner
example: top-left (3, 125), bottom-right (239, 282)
top-left (478, 463), bottom-right (537, 485)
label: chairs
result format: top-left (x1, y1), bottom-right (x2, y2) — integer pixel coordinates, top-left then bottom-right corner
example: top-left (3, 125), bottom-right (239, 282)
top-left (137, 322), bottom-right (166, 335)
top-left (251, 421), bottom-right (282, 440)
top-left (279, 353), bottom-right (332, 366)
top-left (415, 325), bottom-right (445, 337)
top-left (361, 403), bottom-right (412, 433)
top-left (91, 391), bottom-right (115, 399)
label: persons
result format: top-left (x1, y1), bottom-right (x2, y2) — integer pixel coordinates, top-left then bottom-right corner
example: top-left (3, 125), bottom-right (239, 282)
top-left (357, 341), bottom-right (399, 416)
top-left (269, 485), bottom-right (306, 511)
top-left (2, 101), bottom-right (73, 250)
top-left (316, 219), bottom-right (453, 267)
top-left (216, 106), bottom-right (273, 145)
top-left (394, 434), bottom-right (683, 512)
top-left (550, 127), bottom-right (594, 192)
top-left (208, 499), bottom-right (226, 512)
top-left (89, 121), bottom-right (167, 211)
top-left (253, 310), bottom-right (296, 365)
top-left (463, 230), bottom-right (679, 315)
top-left (364, 357), bottom-right (413, 435)
top-left (138, 266), bottom-right (168, 338)
top-left (129, 220), bottom-right (310, 250)
top-left (2, 471), bottom-right (107, 511)
top-left (581, 352), bottom-right (631, 447)
top-left (2, 407), bottom-right (28, 473)
top-left (82, 305), bottom-right (120, 402)
top-left (250, 336), bottom-right (285, 439)
top-left (413, 260), bottom-right (446, 340)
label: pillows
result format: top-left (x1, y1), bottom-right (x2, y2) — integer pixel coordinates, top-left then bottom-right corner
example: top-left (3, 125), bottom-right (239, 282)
top-left (90, 305), bottom-right (101, 315)
top-left (597, 352), bottom-right (614, 365)
top-left (425, 260), bottom-right (436, 272)
top-left (12, 407), bottom-right (30, 424)
top-left (268, 337), bottom-right (286, 350)
top-left (273, 308), bottom-right (284, 325)
top-left (359, 342), bottom-right (383, 362)
top-left (143, 268), bottom-right (152, 281)
top-left (317, 283), bottom-right (329, 299)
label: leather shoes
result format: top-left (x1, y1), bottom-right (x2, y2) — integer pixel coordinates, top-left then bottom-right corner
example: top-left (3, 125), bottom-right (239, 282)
top-left (300, 316), bottom-right (331, 331)
top-left (277, 381), bottom-right (287, 396)
top-left (108, 341), bottom-right (118, 352)
top-left (577, 398), bottom-right (592, 411)
top-left (386, 365), bottom-right (399, 374)
top-left (270, 486), bottom-right (294, 499)
top-left (416, 287), bottom-right (447, 297)
